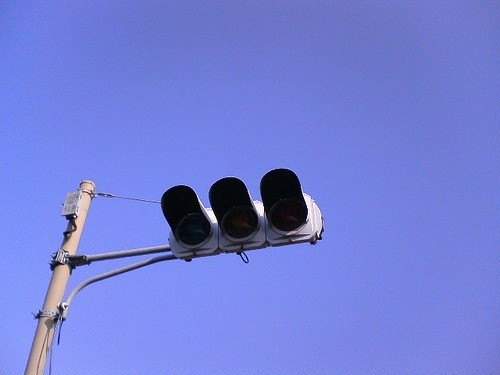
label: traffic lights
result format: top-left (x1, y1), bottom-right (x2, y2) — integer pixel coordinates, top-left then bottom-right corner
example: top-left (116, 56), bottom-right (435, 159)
top-left (160, 167), bottom-right (325, 260)
